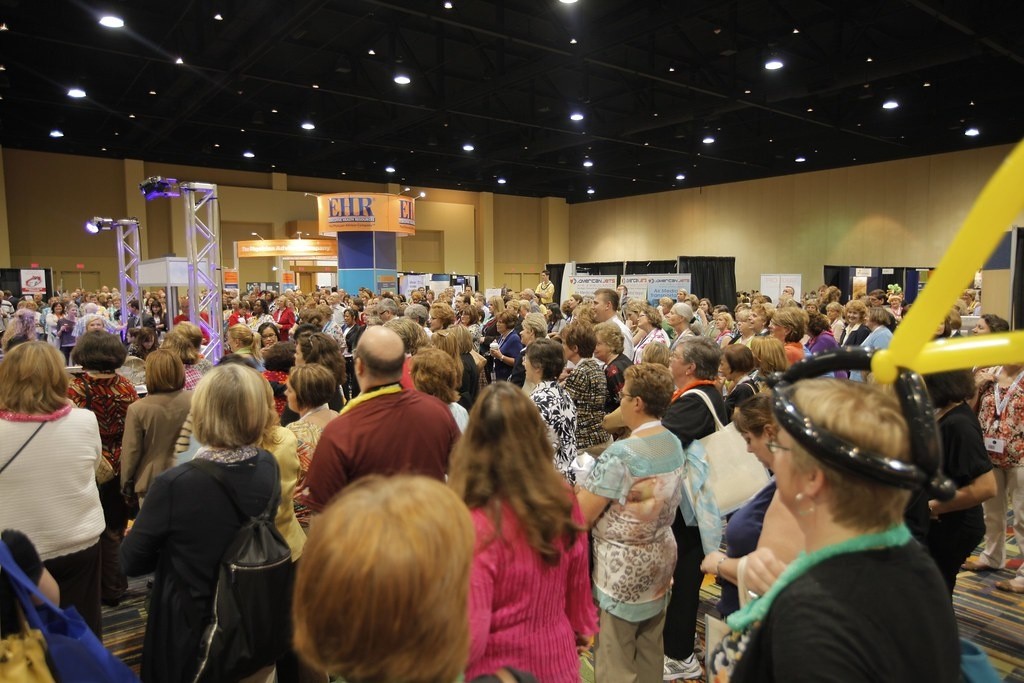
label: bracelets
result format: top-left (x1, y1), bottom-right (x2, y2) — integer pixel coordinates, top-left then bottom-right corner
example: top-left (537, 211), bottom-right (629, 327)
top-left (499, 355), bottom-right (504, 361)
top-left (716, 557), bottom-right (726, 575)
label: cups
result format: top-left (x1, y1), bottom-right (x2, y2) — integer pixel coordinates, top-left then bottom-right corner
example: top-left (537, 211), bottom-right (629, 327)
top-left (490, 343), bottom-right (499, 350)
top-left (64, 324), bottom-right (69, 332)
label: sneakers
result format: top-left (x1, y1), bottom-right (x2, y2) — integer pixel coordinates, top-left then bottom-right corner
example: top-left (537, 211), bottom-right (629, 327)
top-left (663, 653), bottom-right (703, 680)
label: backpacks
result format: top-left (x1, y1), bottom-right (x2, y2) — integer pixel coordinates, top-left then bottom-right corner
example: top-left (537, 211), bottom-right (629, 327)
top-left (185, 449), bottom-right (294, 683)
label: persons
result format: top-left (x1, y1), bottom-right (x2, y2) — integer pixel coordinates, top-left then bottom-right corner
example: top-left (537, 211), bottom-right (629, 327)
top-left (299, 325), bottom-right (463, 530)
top-left (119, 349), bottom-right (193, 538)
top-left (522, 337), bottom-right (578, 487)
top-left (661, 336), bottom-right (727, 681)
top-left (227, 322), bottom-right (267, 372)
top-left (408, 348), bottom-right (469, 434)
top-left (556, 319), bottom-right (613, 459)
top-left (591, 319), bottom-right (634, 441)
top-left (969, 313), bottom-right (1010, 336)
top-left (293, 470), bottom-right (536, 683)
top-left (489, 310), bottom-right (522, 382)
top-left (446, 380), bottom-right (598, 683)
top-left (967, 365), bottom-right (1024, 593)
top-left (576, 364), bottom-right (685, 683)
top-left (900, 367), bottom-right (999, 601)
top-left (0, 270), bottom-right (980, 683)
top-left (283, 363), bottom-right (340, 528)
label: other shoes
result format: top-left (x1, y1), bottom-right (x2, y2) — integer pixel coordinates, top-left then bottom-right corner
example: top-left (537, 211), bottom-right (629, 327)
top-left (147, 577), bottom-right (155, 586)
top-left (101, 598), bottom-right (119, 607)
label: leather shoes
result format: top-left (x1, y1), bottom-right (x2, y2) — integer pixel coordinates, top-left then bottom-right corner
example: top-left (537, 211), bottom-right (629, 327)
top-left (995, 579), bottom-right (1024, 593)
top-left (961, 561), bottom-right (1000, 570)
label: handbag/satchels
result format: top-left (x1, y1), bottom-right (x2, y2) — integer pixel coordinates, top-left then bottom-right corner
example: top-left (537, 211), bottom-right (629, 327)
top-left (679, 388), bottom-right (772, 526)
top-left (705, 556), bottom-right (747, 683)
top-left (53, 333), bottom-right (61, 350)
top-left (0, 540), bottom-right (139, 683)
top-left (71, 374), bottom-right (115, 485)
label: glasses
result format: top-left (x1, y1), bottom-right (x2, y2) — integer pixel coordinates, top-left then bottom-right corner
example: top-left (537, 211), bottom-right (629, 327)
top-left (431, 317), bottom-right (438, 320)
top-left (262, 333), bottom-right (277, 338)
top-left (619, 389), bottom-right (631, 398)
top-left (766, 437), bottom-right (791, 455)
top-left (378, 310), bottom-right (386, 316)
